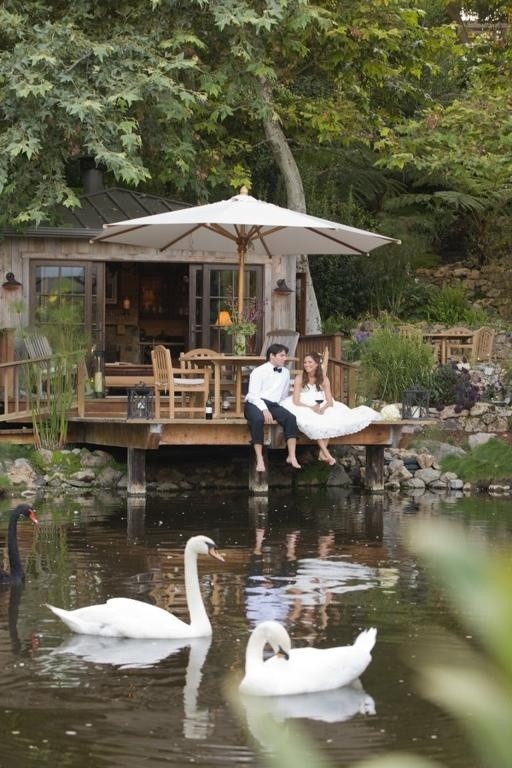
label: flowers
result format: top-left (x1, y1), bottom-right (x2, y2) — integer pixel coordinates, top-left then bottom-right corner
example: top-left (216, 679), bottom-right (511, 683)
top-left (218, 284), bottom-right (268, 338)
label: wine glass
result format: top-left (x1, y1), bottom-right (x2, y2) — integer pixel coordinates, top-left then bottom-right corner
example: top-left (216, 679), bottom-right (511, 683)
top-left (314, 393), bottom-right (325, 410)
top-left (221, 401), bottom-right (231, 422)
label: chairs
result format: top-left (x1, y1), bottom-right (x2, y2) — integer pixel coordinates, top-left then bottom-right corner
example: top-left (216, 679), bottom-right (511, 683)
top-left (151, 329), bottom-right (331, 418)
top-left (19, 325), bottom-right (94, 402)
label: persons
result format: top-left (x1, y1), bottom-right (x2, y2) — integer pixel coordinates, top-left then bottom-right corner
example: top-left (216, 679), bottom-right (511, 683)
top-left (278, 350), bottom-right (380, 468)
top-left (242, 525), bottom-right (300, 655)
top-left (295, 529), bottom-right (379, 650)
top-left (243, 342), bottom-right (302, 474)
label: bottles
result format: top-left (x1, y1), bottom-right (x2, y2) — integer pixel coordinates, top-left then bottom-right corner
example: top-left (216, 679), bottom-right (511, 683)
top-left (122, 294), bottom-right (130, 316)
top-left (132, 395), bottom-right (149, 418)
top-left (204, 393), bottom-right (213, 420)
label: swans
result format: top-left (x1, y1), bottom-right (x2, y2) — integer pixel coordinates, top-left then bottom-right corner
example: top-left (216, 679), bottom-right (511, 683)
top-left (239, 621), bottom-right (378, 696)
top-left (0, 504), bottom-right (38, 585)
top-left (42, 535), bottom-right (225, 639)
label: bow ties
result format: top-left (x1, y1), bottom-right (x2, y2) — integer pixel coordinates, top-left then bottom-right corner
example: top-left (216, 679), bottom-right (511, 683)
top-left (273, 367), bottom-right (282, 373)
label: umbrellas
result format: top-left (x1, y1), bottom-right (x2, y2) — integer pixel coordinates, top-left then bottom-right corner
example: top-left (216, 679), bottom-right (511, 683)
top-left (87, 185), bottom-right (403, 412)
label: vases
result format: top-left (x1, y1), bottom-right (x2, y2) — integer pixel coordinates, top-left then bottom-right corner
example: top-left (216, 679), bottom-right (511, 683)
top-left (235, 331), bottom-right (246, 354)
